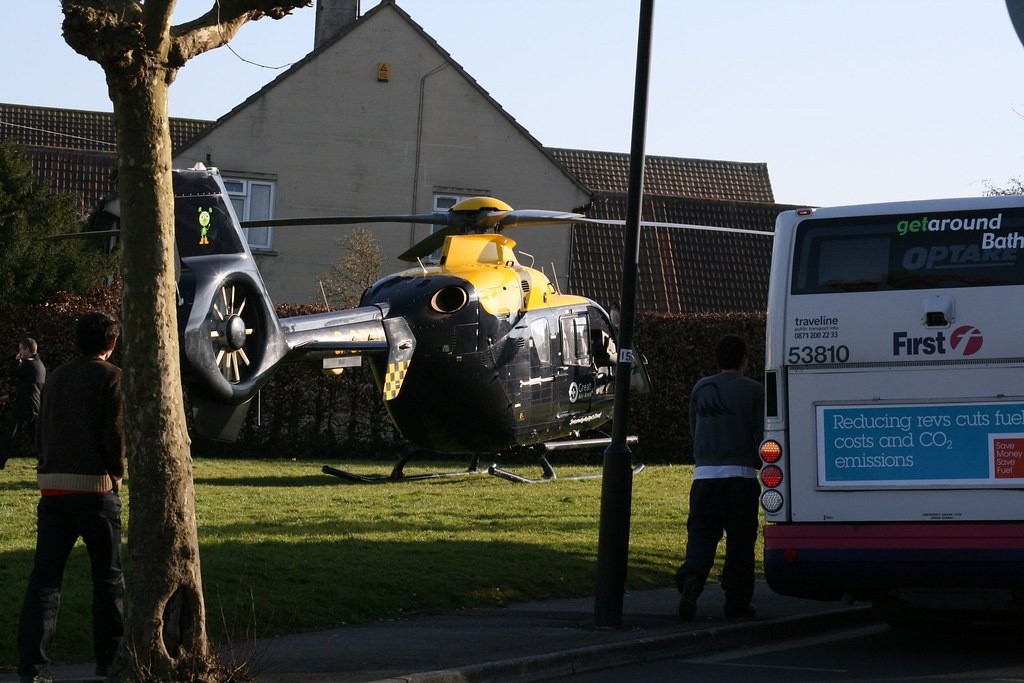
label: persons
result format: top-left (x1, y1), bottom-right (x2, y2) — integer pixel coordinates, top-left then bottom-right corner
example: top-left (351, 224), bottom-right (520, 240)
top-left (17, 311), bottom-right (126, 683)
top-left (674, 336), bottom-right (766, 623)
top-left (0, 338), bottom-right (46, 470)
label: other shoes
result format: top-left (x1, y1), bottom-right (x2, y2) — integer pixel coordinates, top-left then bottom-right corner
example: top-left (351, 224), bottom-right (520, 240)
top-left (21, 672), bottom-right (54, 683)
top-left (95, 654), bottom-right (112, 676)
top-left (674, 578), bottom-right (697, 621)
top-left (726, 599), bottom-right (755, 619)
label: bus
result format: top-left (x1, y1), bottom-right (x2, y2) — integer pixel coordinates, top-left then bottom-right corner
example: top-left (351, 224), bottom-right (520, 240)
top-left (758, 193), bottom-right (1024, 597)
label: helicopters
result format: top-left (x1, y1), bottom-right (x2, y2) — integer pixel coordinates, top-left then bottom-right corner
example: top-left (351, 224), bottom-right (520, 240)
top-left (25, 160), bottom-right (776, 486)
